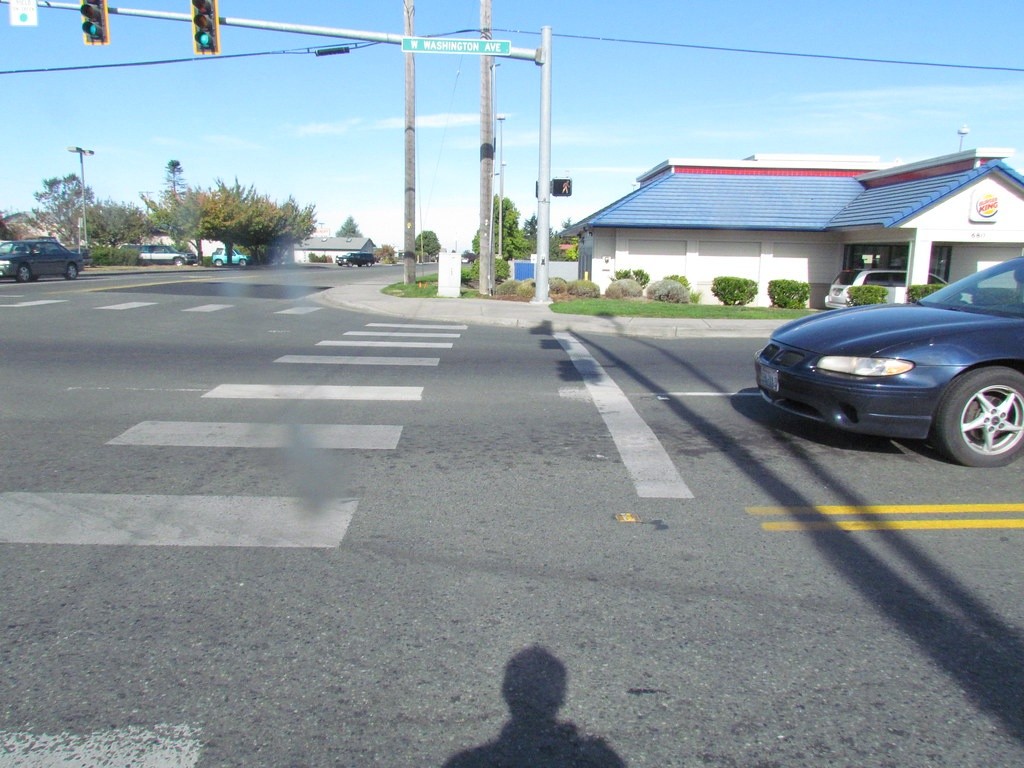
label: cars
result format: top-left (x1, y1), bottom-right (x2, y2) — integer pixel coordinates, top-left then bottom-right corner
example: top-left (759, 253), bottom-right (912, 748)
top-left (117, 243), bottom-right (197, 266)
top-left (754, 256), bottom-right (1024, 467)
top-left (0, 240), bottom-right (84, 283)
top-left (825, 270), bottom-right (950, 309)
top-left (211, 248), bottom-right (254, 267)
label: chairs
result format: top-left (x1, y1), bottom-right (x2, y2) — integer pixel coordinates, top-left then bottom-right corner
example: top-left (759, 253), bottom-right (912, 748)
top-left (50, 249), bottom-right (60, 256)
top-left (16, 247), bottom-right (28, 251)
top-left (39, 248), bottom-right (48, 256)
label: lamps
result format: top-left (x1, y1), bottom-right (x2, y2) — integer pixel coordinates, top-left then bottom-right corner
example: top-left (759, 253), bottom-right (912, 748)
top-left (938, 258), bottom-right (944, 265)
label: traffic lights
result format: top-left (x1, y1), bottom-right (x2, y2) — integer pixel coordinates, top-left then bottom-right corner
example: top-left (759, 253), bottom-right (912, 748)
top-left (551, 179), bottom-right (573, 196)
top-left (80, 0), bottom-right (110, 45)
top-left (190, 0), bottom-right (221, 56)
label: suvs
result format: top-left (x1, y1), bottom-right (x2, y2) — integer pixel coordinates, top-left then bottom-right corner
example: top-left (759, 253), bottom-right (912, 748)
top-left (336, 253), bottom-right (375, 267)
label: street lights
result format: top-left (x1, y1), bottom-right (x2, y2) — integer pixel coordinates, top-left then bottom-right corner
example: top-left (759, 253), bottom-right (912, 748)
top-left (496, 114), bottom-right (506, 257)
top-left (68, 147), bottom-right (93, 249)
